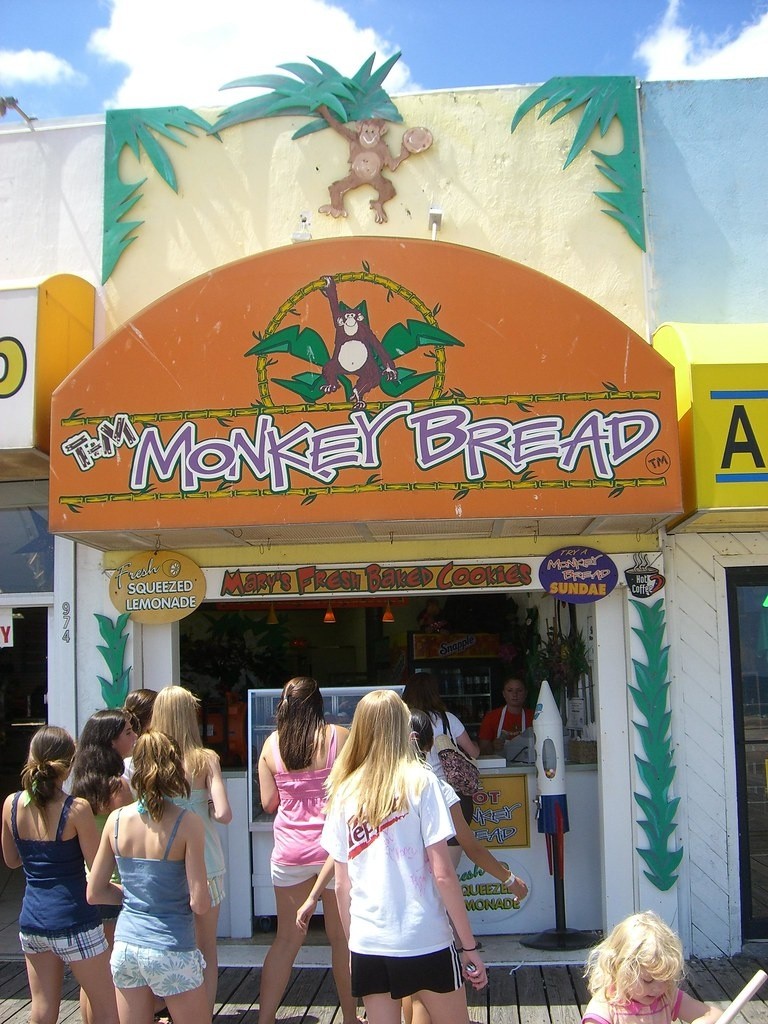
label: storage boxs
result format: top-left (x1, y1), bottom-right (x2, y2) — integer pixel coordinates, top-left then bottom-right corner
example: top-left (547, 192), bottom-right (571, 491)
top-left (569, 740), bottom-right (597, 763)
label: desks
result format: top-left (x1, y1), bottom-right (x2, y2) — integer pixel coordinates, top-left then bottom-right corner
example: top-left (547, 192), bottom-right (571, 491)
top-left (455, 761), bottom-right (603, 935)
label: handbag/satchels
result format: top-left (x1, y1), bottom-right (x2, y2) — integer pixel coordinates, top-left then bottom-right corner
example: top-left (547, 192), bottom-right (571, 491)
top-left (434, 711), bottom-right (480, 796)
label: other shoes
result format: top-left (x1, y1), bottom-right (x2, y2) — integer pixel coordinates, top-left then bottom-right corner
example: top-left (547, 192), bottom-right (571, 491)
top-left (152, 1006), bottom-right (173, 1024)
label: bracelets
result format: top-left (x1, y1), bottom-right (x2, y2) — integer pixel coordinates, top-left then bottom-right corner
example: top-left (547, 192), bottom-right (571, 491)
top-left (503, 870), bottom-right (517, 887)
top-left (461, 946), bottom-right (477, 952)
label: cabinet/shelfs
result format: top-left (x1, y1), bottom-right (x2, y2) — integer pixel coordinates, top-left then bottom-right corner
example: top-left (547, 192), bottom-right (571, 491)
top-left (247, 684), bottom-right (407, 930)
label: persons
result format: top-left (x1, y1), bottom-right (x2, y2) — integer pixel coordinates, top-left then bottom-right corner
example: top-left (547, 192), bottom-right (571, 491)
top-left (261, 672), bottom-right (528, 1024)
top-left (580, 909), bottom-right (725, 1024)
top-left (478, 676), bottom-right (537, 755)
top-left (0, 685), bottom-right (233, 1023)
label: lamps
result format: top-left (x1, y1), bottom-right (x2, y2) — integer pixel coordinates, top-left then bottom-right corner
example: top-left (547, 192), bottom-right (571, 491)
top-left (383, 597), bottom-right (396, 622)
top-left (266, 602), bottom-right (279, 625)
top-left (324, 599), bottom-right (335, 623)
